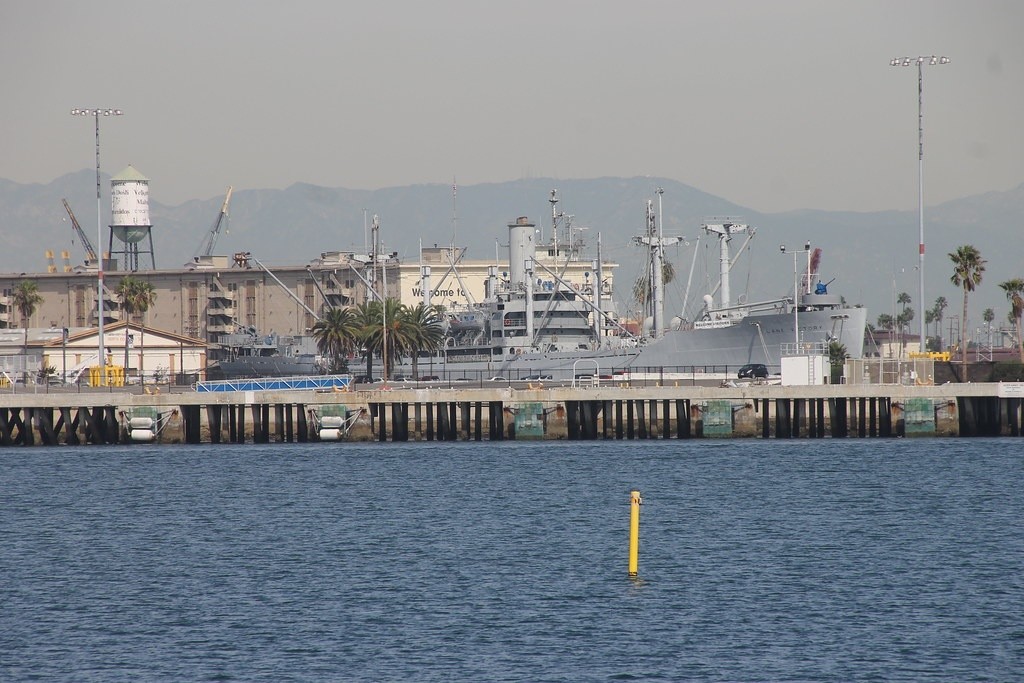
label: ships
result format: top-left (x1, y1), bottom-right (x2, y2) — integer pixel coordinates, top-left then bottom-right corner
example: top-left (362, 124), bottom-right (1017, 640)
top-left (217, 184), bottom-right (866, 381)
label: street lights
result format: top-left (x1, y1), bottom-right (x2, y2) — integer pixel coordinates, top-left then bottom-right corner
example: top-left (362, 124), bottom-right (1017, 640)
top-left (890, 54), bottom-right (951, 356)
top-left (70, 108), bottom-right (124, 386)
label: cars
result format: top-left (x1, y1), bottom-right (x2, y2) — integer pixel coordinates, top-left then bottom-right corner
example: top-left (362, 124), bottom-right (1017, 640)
top-left (738, 363), bottom-right (770, 379)
top-left (354, 376), bottom-right (373, 382)
top-left (486, 377), bottom-right (507, 380)
